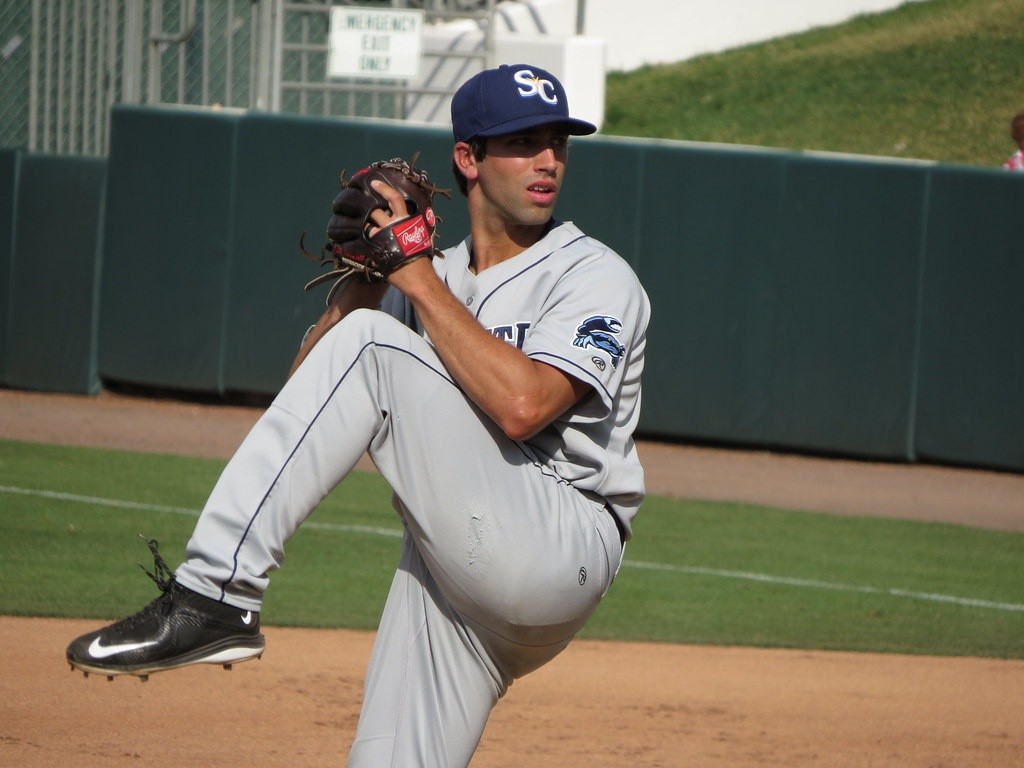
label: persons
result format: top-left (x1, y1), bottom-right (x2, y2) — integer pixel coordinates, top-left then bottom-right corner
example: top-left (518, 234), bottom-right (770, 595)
top-left (1000, 116), bottom-right (1024, 169)
top-left (66, 66), bottom-right (651, 768)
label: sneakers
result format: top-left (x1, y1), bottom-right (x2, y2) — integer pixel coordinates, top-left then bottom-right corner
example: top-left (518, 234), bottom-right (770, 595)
top-left (66, 539), bottom-right (265, 678)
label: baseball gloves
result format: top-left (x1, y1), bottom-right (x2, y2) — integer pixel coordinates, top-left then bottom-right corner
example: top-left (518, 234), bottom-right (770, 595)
top-left (296, 150), bottom-right (453, 307)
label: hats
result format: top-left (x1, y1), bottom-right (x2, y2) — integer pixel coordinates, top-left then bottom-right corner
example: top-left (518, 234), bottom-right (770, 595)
top-left (451, 62), bottom-right (596, 142)
